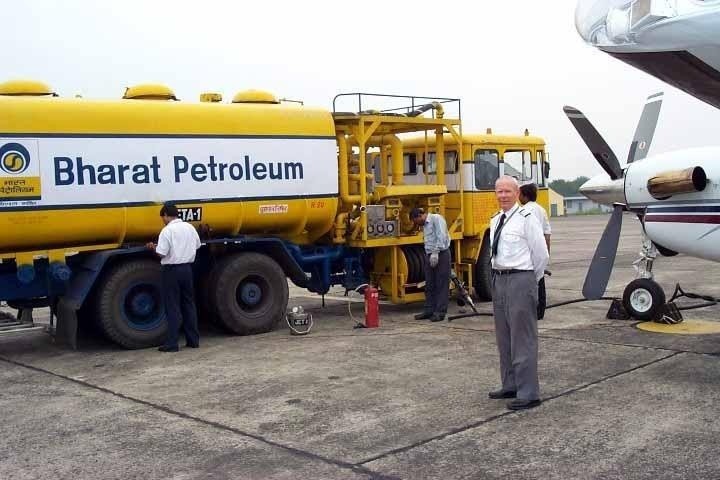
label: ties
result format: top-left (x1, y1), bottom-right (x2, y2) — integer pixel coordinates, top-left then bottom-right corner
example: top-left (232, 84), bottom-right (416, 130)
top-left (492, 213), bottom-right (507, 258)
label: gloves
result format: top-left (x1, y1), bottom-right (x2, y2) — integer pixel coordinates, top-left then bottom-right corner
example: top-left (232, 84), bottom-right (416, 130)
top-left (430, 251), bottom-right (440, 267)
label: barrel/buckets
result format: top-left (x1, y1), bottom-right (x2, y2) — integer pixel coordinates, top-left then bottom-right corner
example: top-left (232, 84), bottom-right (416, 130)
top-left (285, 311), bottom-right (314, 336)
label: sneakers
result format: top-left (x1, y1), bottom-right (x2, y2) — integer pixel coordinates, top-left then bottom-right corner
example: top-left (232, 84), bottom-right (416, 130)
top-left (414, 311), bottom-right (444, 323)
top-left (156, 341), bottom-right (201, 352)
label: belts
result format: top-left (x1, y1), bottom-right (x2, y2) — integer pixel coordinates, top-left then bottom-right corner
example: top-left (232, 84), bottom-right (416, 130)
top-left (492, 267), bottom-right (536, 277)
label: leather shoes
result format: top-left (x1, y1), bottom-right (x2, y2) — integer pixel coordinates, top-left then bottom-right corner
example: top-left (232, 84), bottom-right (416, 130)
top-left (487, 387), bottom-right (517, 398)
top-left (506, 394), bottom-right (541, 411)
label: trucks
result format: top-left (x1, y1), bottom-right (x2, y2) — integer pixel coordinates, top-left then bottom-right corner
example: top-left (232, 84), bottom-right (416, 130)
top-left (0, 81), bottom-right (551, 350)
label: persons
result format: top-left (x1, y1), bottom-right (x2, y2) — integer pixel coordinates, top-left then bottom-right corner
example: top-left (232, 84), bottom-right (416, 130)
top-left (488, 174), bottom-right (551, 409)
top-left (145, 203), bottom-right (202, 353)
top-left (519, 183), bottom-right (552, 320)
top-left (408, 208), bottom-right (452, 321)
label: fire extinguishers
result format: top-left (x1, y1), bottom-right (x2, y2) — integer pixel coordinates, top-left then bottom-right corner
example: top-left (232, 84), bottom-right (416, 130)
top-left (364, 283), bottom-right (380, 328)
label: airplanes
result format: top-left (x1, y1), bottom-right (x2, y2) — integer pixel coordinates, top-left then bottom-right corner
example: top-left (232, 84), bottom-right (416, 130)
top-left (562, 0), bottom-right (719, 320)
